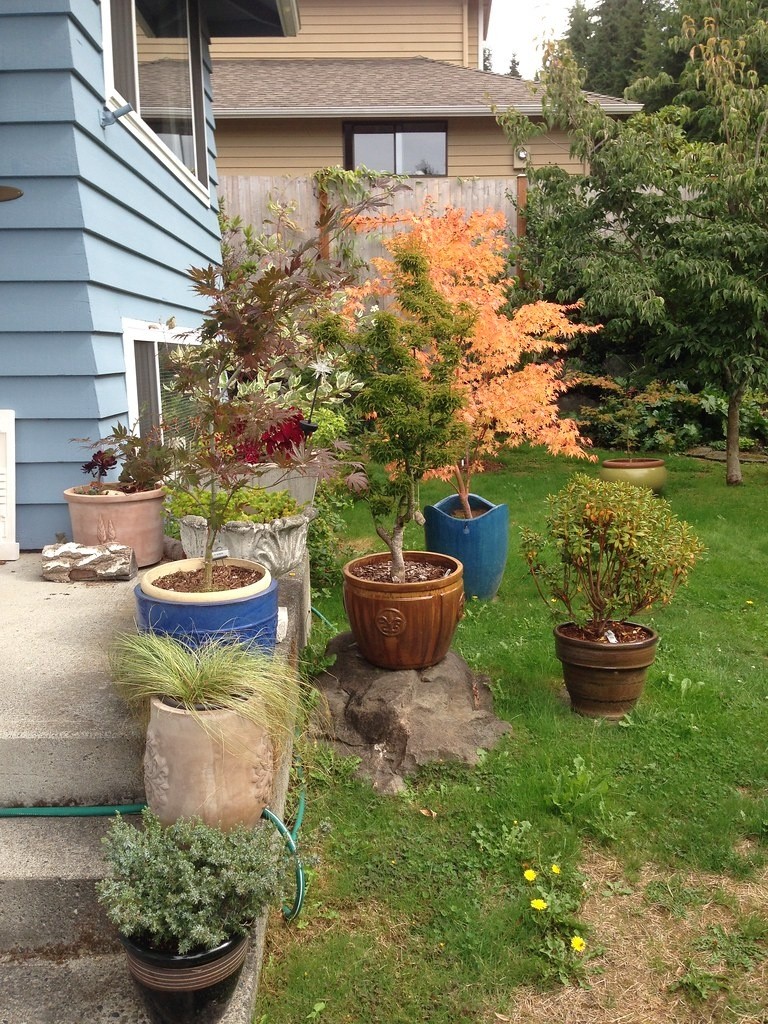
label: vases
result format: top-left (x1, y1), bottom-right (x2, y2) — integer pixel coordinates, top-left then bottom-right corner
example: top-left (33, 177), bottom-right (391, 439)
top-left (63, 482), bottom-right (167, 568)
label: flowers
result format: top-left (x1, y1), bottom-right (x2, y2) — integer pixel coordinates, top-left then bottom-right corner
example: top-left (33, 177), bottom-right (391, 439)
top-left (75, 451), bottom-right (146, 493)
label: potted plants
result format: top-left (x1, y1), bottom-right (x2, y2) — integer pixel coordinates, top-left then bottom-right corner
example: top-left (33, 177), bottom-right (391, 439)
top-left (111, 609), bottom-right (321, 840)
top-left (339, 191), bottom-right (602, 602)
top-left (160, 486), bottom-right (318, 575)
top-left (93, 805), bottom-right (284, 1024)
top-left (576, 371), bottom-right (699, 495)
top-left (305, 245), bottom-right (480, 669)
top-left (131, 202), bottom-right (354, 660)
top-left (519, 474), bottom-right (711, 719)
